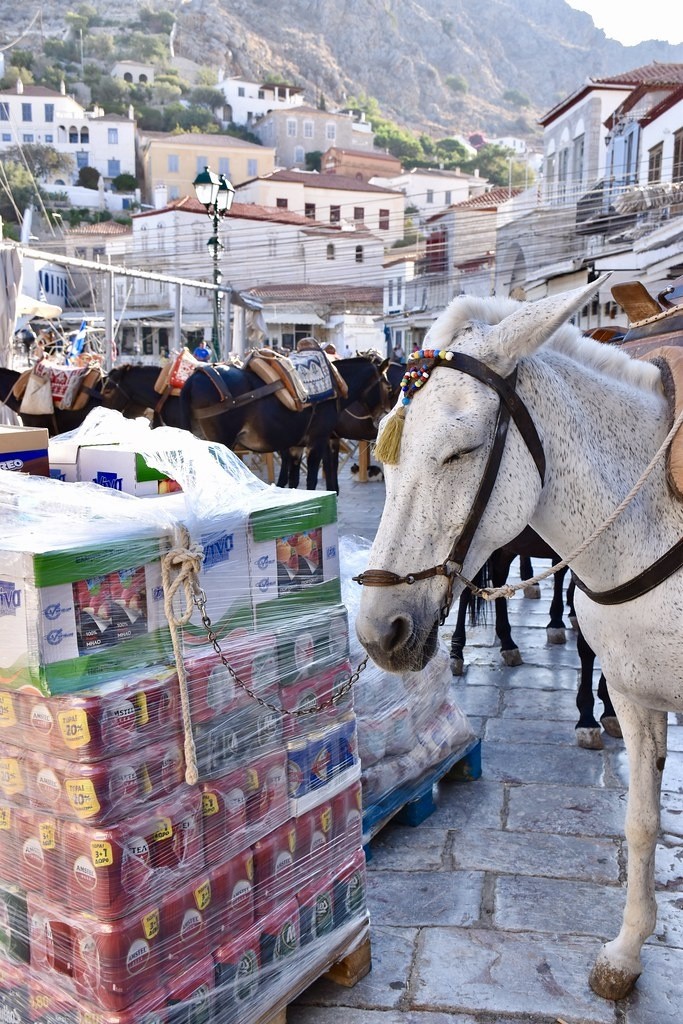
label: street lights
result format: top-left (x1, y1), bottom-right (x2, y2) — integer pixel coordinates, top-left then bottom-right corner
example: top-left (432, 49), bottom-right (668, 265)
top-left (191, 165), bottom-right (237, 364)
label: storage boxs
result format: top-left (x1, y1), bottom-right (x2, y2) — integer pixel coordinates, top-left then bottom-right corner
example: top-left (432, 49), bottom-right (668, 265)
top-left (0, 426), bottom-right (368, 1024)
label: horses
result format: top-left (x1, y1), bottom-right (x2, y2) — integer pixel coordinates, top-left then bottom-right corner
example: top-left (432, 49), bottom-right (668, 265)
top-left (356, 275), bottom-right (682, 1001)
top-left (0, 350), bottom-right (624, 754)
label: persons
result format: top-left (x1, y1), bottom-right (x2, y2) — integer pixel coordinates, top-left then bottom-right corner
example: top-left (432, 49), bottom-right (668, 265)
top-left (193, 339), bottom-right (211, 363)
top-left (33, 329), bottom-right (57, 363)
top-left (391, 342), bottom-right (406, 364)
top-left (412, 342), bottom-right (419, 353)
